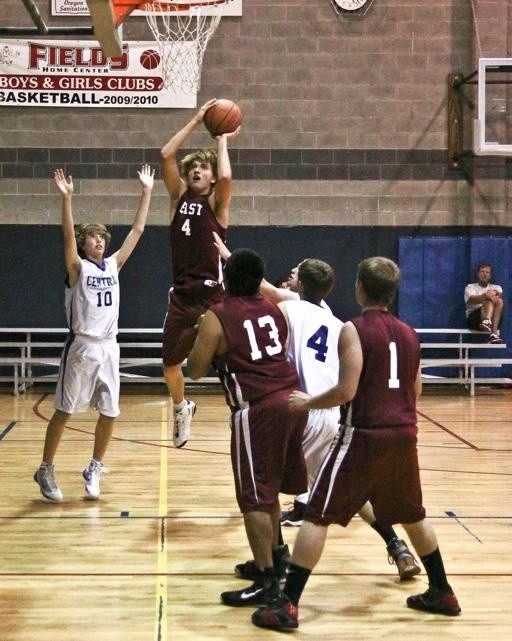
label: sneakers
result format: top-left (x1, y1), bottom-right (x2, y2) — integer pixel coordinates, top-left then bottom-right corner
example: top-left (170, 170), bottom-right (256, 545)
top-left (172, 399), bottom-right (197, 447)
top-left (386, 537), bottom-right (421, 581)
top-left (279, 500), bottom-right (307, 527)
top-left (34, 465), bottom-right (63, 503)
top-left (83, 460), bottom-right (104, 499)
top-left (234, 544), bottom-right (290, 583)
top-left (252, 593), bottom-right (298, 628)
top-left (479, 320), bottom-right (492, 331)
top-left (489, 334), bottom-right (501, 344)
top-left (407, 587), bottom-right (461, 616)
top-left (220, 578), bottom-right (281, 608)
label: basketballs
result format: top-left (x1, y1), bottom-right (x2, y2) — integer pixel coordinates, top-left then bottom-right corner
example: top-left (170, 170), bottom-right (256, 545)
top-left (140, 50), bottom-right (160, 70)
top-left (203, 99), bottom-right (242, 136)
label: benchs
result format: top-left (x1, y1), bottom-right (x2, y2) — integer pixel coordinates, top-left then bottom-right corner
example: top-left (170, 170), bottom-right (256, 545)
top-left (1, 326), bottom-right (512, 398)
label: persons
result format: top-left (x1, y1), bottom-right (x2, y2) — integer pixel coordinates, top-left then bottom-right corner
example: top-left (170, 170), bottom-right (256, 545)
top-left (187, 249), bottom-right (308, 608)
top-left (218, 256), bottom-right (422, 610)
top-left (211, 229), bottom-right (335, 529)
top-left (248, 257), bottom-right (462, 629)
top-left (462, 261), bottom-right (506, 345)
top-left (34, 161), bottom-right (158, 503)
top-left (160, 98), bottom-right (239, 449)
